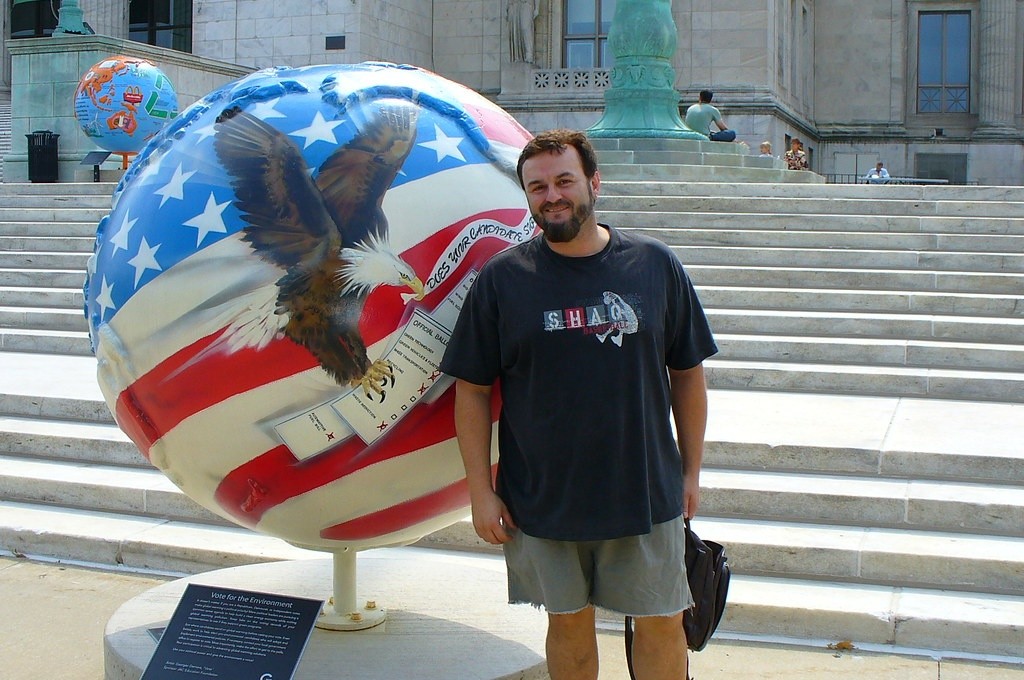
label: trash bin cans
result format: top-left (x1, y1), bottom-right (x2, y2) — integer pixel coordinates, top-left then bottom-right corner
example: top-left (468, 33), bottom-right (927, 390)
top-left (24, 130), bottom-right (60, 183)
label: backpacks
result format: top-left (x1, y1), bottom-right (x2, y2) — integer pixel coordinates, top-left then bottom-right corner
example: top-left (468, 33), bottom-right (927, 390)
top-left (682, 515), bottom-right (730, 652)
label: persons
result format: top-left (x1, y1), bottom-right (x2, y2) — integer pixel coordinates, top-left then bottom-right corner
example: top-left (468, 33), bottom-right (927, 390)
top-left (437, 128), bottom-right (720, 680)
top-left (759, 142), bottom-right (773, 157)
top-left (866, 162), bottom-right (890, 184)
top-left (783, 138), bottom-right (808, 171)
top-left (686, 89), bottom-right (736, 142)
